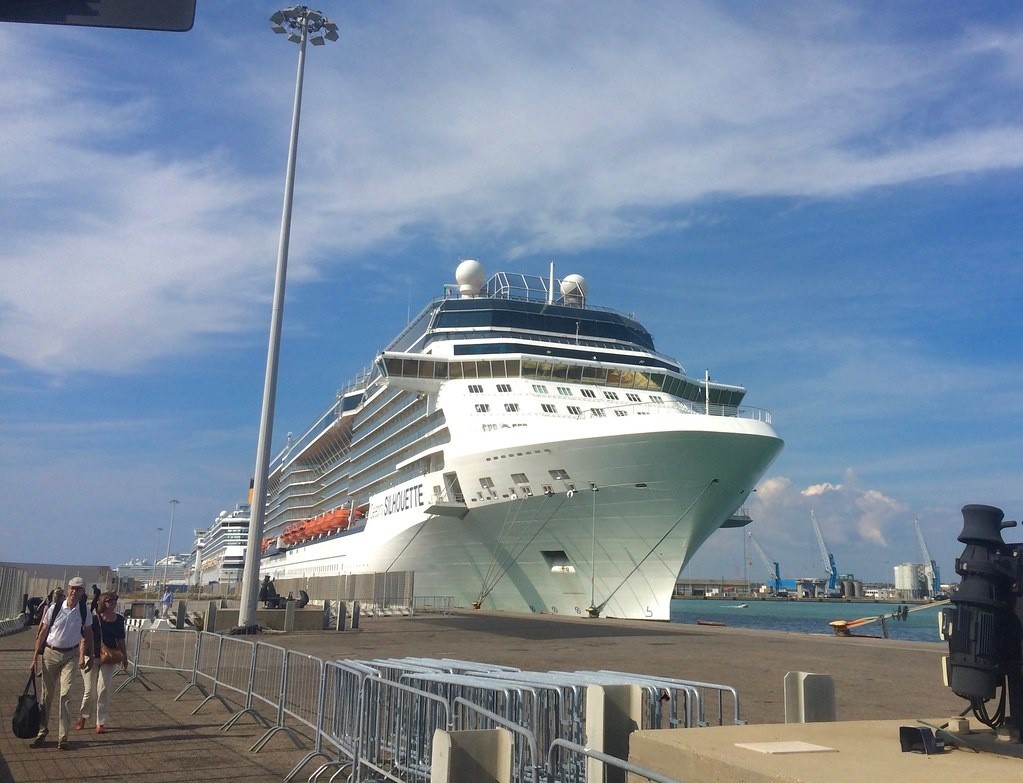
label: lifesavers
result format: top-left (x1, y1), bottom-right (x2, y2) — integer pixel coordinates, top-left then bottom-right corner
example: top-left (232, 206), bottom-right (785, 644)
top-left (697, 621), bottom-right (725, 626)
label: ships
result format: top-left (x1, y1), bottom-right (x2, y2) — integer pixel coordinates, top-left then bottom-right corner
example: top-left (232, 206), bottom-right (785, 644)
top-left (257, 259), bottom-right (786, 624)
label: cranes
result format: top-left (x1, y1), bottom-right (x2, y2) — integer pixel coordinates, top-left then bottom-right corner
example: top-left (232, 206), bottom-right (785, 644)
top-left (747, 531), bottom-right (781, 598)
top-left (914, 517), bottom-right (940, 603)
top-left (810, 508), bottom-right (838, 598)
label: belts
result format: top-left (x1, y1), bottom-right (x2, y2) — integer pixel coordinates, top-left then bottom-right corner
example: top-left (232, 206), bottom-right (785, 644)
top-left (46, 643), bottom-right (79, 652)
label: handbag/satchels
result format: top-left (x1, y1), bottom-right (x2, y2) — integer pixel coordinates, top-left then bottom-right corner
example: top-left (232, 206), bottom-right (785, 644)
top-left (100, 642), bottom-right (122, 664)
top-left (11, 668), bottom-right (41, 739)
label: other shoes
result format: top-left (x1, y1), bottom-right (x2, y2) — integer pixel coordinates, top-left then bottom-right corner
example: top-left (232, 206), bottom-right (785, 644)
top-left (58, 743), bottom-right (68, 750)
top-left (34, 735), bottom-right (47, 746)
top-left (76, 721), bottom-right (84, 730)
top-left (96, 725), bottom-right (104, 734)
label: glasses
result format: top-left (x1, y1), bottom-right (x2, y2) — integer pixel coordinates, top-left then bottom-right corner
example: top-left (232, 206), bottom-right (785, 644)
top-left (105, 598), bottom-right (117, 603)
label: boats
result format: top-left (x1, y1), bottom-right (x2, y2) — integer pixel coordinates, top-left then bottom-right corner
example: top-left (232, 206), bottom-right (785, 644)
top-left (321, 512), bottom-right (339, 534)
top-left (304, 519), bottom-right (320, 538)
top-left (329, 508), bottom-right (362, 529)
top-left (313, 516), bottom-right (327, 535)
top-left (260, 519), bottom-right (305, 554)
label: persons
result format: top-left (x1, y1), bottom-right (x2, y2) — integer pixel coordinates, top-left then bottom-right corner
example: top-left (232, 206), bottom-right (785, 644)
top-left (76, 591), bottom-right (128, 735)
top-left (90, 585), bottom-right (101, 614)
top-left (160, 586), bottom-right (173, 619)
top-left (29, 577), bottom-right (94, 750)
top-left (36, 587), bottom-right (66, 677)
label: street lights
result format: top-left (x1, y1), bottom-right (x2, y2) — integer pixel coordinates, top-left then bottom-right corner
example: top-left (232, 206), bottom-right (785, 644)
top-left (161, 499), bottom-right (181, 588)
top-left (151, 527), bottom-right (165, 591)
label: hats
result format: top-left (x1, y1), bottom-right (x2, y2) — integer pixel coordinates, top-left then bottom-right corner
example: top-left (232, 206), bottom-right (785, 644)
top-left (68, 577), bottom-right (86, 589)
